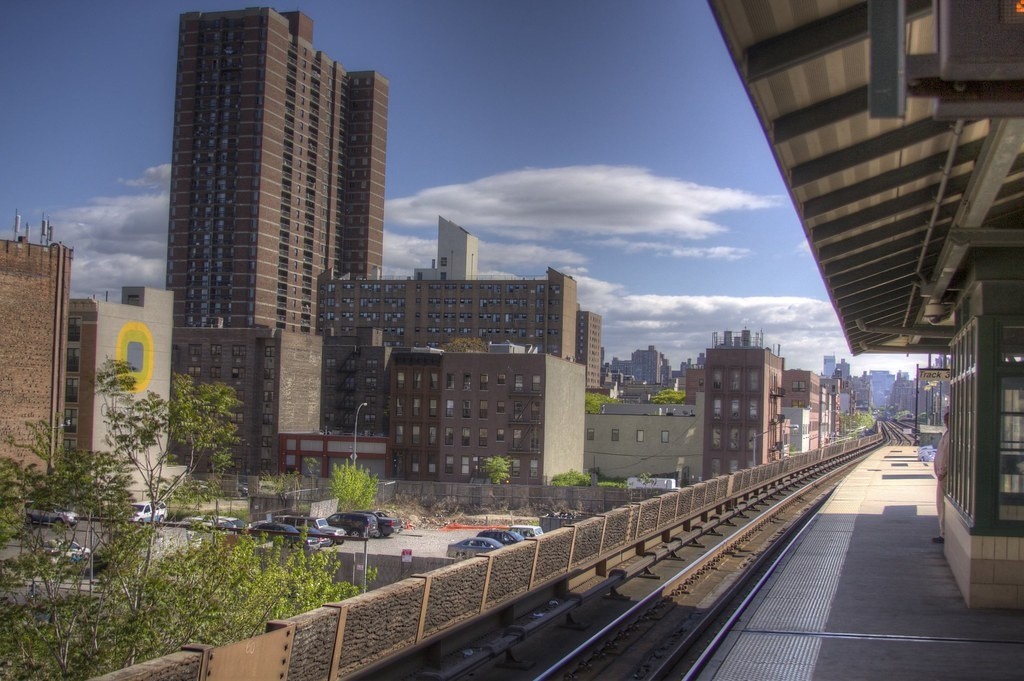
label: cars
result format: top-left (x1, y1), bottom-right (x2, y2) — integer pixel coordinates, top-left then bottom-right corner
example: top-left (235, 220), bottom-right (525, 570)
top-left (509, 525), bottom-right (543, 537)
top-left (35, 541), bottom-right (92, 558)
top-left (181, 514), bottom-right (247, 534)
top-left (446, 538), bottom-right (503, 561)
top-left (248, 524), bottom-right (320, 549)
top-left (358, 511), bottom-right (402, 538)
top-left (476, 530), bottom-right (525, 547)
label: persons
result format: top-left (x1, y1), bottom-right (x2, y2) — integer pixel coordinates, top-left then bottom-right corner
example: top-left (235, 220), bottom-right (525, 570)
top-left (932, 412), bottom-right (948, 543)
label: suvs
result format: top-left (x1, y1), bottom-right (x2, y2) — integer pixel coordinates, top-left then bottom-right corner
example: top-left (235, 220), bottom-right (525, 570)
top-left (324, 513), bottom-right (378, 539)
top-left (116, 503), bottom-right (169, 524)
top-left (285, 517), bottom-right (348, 544)
top-left (21, 502), bottom-right (80, 526)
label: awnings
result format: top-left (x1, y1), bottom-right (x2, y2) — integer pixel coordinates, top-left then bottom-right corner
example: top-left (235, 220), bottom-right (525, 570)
top-left (704, 0), bottom-right (1022, 356)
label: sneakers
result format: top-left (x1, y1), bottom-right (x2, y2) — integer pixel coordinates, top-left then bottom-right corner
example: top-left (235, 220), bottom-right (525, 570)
top-left (932, 536), bottom-right (944, 542)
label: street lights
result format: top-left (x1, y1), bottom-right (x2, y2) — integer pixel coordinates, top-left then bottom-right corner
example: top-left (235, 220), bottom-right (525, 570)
top-left (752, 425), bottom-right (799, 467)
top-left (352, 402), bottom-right (368, 469)
